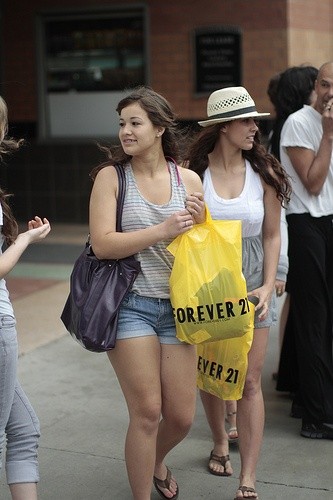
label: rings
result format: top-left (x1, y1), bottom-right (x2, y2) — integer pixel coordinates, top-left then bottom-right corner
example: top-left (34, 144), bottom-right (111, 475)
top-left (186, 221), bottom-right (188, 226)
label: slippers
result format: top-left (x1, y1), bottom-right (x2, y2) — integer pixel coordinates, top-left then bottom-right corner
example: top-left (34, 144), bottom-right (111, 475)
top-left (233, 485), bottom-right (258, 500)
top-left (208, 449), bottom-right (233, 476)
top-left (152, 464), bottom-right (179, 500)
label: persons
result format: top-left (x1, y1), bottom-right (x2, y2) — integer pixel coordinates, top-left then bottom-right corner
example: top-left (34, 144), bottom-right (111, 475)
top-left (226, 400), bottom-right (239, 439)
top-left (177, 86), bottom-right (294, 500)
top-left (89, 85), bottom-right (206, 500)
top-left (0, 96), bottom-right (51, 500)
top-left (266, 61), bottom-right (333, 439)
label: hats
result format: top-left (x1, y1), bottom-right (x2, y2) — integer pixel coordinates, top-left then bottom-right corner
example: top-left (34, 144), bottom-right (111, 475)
top-left (198, 86), bottom-right (270, 128)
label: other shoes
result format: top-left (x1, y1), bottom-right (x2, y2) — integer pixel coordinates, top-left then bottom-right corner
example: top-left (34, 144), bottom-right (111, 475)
top-left (299, 419), bottom-right (333, 440)
top-left (226, 427), bottom-right (239, 443)
top-left (290, 407), bottom-right (303, 419)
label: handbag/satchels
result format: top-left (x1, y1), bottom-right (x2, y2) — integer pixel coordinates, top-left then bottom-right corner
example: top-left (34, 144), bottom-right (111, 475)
top-left (165, 192), bottom-right (254, 346)
top-left (60, 163), bottom-right (141, 353)
top-left (196, 300), bottom-right (255, 401)
